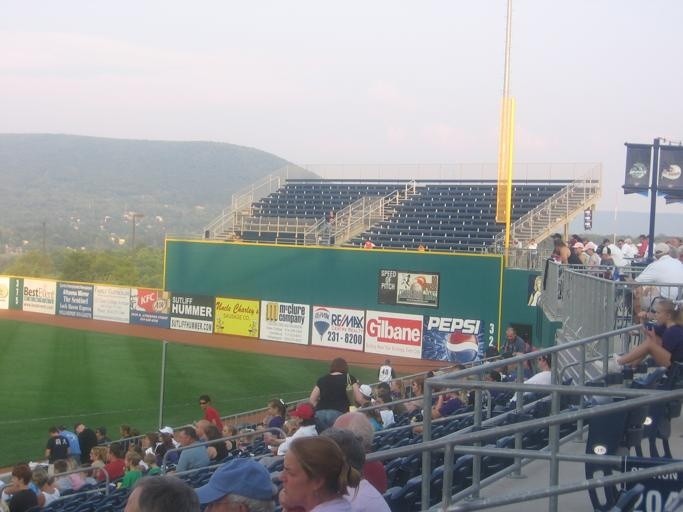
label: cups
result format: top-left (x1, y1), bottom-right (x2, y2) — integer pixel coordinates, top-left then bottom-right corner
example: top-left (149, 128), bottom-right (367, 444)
top-left (645, 321), bottom-right (656, 330)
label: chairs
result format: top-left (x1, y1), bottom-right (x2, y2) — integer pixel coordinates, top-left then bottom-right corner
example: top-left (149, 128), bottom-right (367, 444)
top-left (2, 365), bottom-right (577, 510)
top-left (577, 253), bottom-right (681, 511)
top-left (232, 182), bottom-right (572, 253)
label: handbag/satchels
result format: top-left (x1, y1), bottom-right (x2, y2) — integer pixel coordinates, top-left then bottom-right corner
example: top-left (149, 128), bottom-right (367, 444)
top-left (345, 373), bottom-right (355, 401)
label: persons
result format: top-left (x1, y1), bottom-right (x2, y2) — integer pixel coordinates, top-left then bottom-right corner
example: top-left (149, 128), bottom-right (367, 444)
top-left (550, 233), bottom-right (683, 391)
top-left (0, 397), bottom-right (392, 512)
top-left (310, 325), bottom-right (551, 433)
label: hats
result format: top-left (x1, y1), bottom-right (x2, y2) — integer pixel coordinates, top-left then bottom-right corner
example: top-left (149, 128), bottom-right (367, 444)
top-left (358, 384), bottom-right (372, 397)
top-left (551, 234), bottom-right (561, 238)
top-left (159, 427), bottom-right (174, 437)
top-left (654, 243), bottom-right (670, 255)
top-left (572, 241), bottom-right (611, 255)
top-left (287, 403), bottom-right (314, 419)
top-left (193, 459), bottom-right (275, 505)
